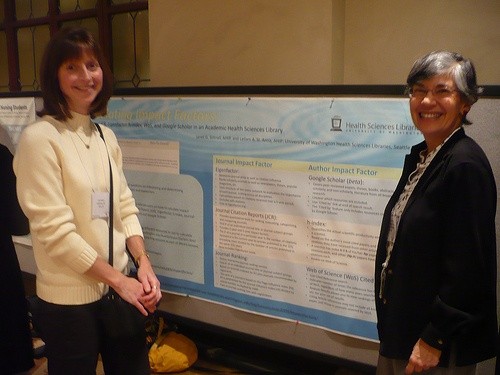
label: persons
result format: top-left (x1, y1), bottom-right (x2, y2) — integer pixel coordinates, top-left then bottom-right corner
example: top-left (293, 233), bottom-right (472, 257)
top-left (12, 29), bottom-right (163, 375)
top-left (1, 143), bottom-right (37, 375)
top-left (366, 49), bottom-right (500, 375)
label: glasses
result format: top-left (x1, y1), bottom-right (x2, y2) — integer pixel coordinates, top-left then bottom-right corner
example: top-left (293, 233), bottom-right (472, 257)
top-left (408, 86), bottom-right (463, 99)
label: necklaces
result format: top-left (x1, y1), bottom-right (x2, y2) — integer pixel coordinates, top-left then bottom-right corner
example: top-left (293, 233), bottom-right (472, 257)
top-left (63, 109), bottom-right (93, 150)
